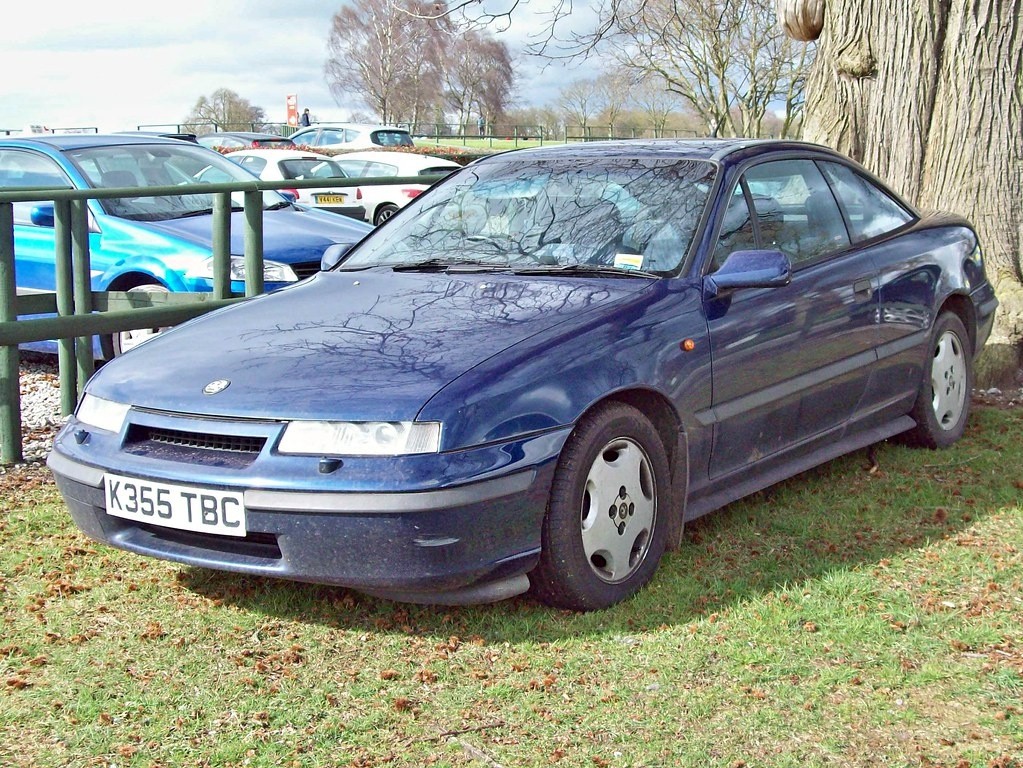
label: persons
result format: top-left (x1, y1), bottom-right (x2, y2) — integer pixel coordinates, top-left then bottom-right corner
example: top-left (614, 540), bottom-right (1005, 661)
top-left (302, 108), bottom-right (310, 127)
top-left (297, 112), bottom-right (300, 126)
top-left (477, 114), bottom-right (485, 139)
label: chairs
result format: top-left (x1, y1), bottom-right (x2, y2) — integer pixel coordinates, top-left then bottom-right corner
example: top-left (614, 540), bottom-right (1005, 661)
top-left (560, 196), bottom-right (621, 245)
top-left (101, 170), bottom-right (155, 215)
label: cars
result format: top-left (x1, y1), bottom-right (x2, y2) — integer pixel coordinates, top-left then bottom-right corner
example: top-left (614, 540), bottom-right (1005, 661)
top-left (105, 118), bottom-right (468, 229)
top-left (51, 137), bottom-right (1001, 612)
top-left (0, 129), bottom-right (418, 368)
